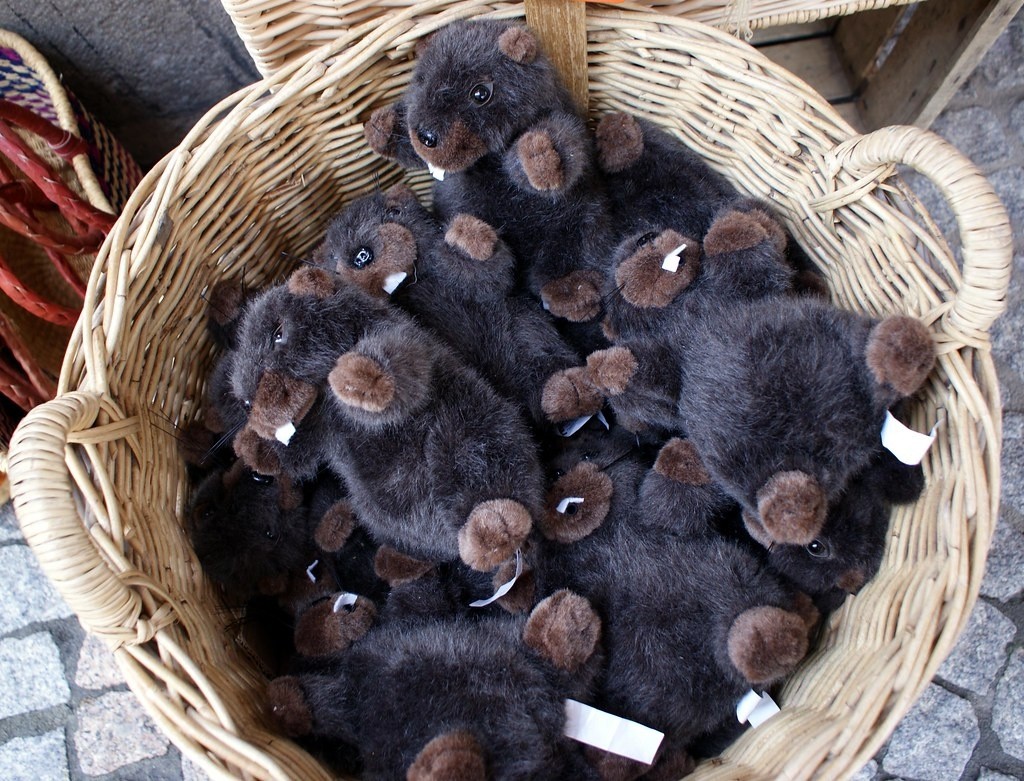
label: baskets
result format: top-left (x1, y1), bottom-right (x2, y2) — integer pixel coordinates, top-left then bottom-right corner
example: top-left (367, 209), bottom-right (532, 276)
top-left (0, 0), bottom-right (1011, 781)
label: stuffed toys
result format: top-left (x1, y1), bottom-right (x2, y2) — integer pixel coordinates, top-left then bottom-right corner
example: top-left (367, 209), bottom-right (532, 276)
top-left (178, 23), bottom-right (937, 781)
